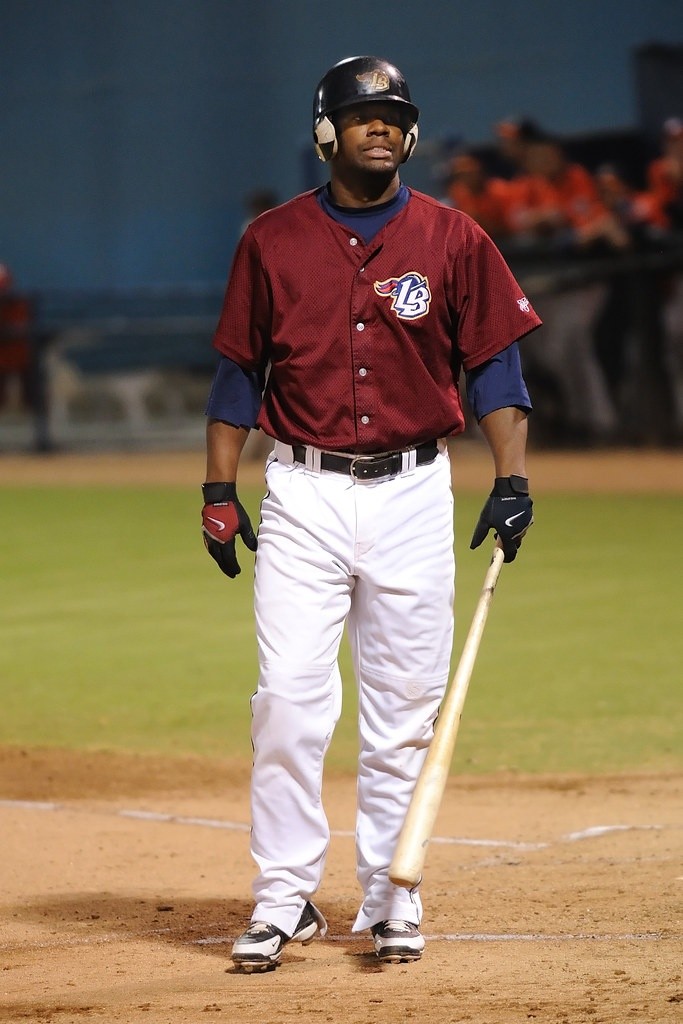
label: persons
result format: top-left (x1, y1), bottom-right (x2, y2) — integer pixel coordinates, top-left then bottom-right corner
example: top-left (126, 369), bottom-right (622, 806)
top-left (241, 116), bottom-right (683, 460)
top-left (202, 56), bottom-right (544, 974)
top-left (0, 263), bottom-right (31, 424)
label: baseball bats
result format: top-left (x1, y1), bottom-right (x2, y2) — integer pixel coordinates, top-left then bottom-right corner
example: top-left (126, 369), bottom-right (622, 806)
top-left (388, 534), bottom-right (504, 889)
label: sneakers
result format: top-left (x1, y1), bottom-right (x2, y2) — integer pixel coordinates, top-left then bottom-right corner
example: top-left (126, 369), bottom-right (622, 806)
top-left (232, 901), bottom-right (319, 972)
top-left (370, 919), bottom-right (425, 964)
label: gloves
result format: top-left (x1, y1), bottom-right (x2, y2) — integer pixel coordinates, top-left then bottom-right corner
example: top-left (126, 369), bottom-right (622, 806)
top-left (470, 474), bottom-right (534, 563)
top-left (201, 482), bottom-right (258, 578)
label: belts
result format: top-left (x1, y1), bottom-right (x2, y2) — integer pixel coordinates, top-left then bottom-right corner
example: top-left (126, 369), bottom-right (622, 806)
top-left (293, 438), bottom-right (439, 482)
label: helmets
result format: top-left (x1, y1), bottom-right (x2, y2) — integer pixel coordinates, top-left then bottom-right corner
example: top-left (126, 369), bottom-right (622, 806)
top-left (312, 56), bottom-right (418, 163)
